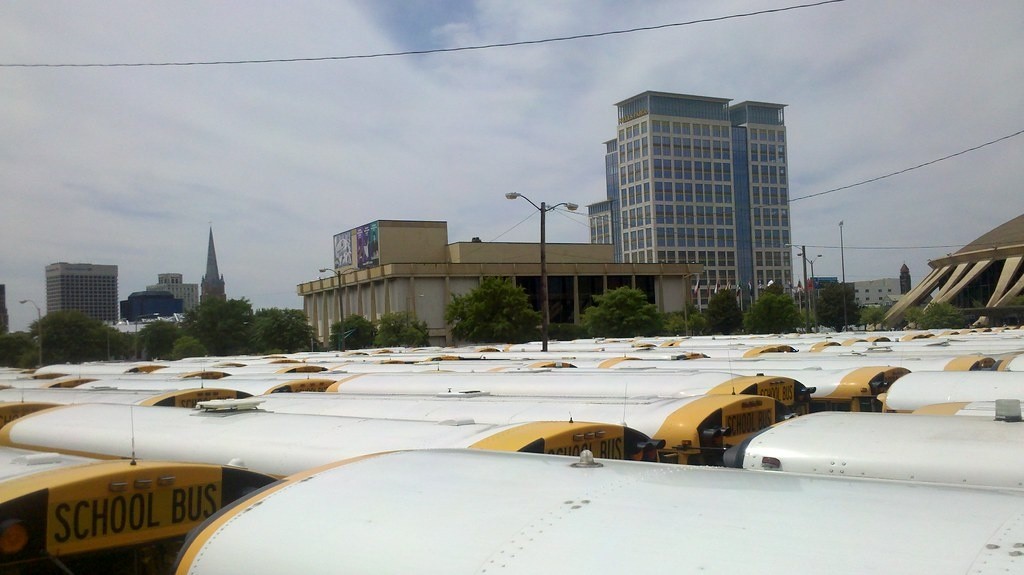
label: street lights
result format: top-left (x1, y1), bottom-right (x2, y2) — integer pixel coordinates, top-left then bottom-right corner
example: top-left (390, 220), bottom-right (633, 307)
top-left (505, 190), bottom-right (579, 349)
top-left (797, 250), bottom-right (823, 332)
top-left (19, 296), bottom-right (44, 368)
top-left (838, 219), bottom-right (851, 332)
top-left (321, 267), bottom-right (357, 351)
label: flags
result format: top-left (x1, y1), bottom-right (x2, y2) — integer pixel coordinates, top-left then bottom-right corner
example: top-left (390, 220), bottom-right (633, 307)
top-left (694, 278), bottom-right (813, 296)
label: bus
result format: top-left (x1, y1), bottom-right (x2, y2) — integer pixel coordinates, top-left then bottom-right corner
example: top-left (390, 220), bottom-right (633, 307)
top-left (0, 326), bottom-right (1024, 572)
